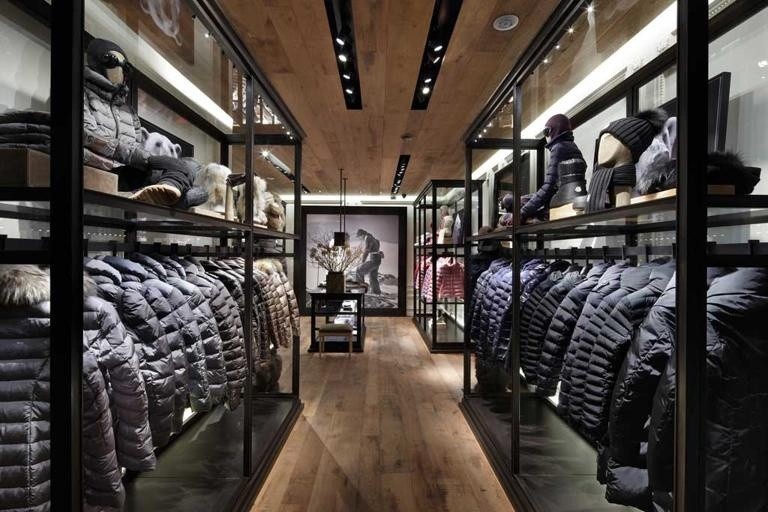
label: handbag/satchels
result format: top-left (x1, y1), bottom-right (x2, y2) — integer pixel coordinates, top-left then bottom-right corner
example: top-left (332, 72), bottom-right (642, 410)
top-left (377, 250), bottom-right (385, 259)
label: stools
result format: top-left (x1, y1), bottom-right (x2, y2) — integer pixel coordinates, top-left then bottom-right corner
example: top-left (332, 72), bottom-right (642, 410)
top-left (318, 324), bottom-right (356, 360)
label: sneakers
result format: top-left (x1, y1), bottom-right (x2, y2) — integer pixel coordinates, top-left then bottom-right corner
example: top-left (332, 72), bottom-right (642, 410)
top-left (126, 183), bottom-right (181, 208)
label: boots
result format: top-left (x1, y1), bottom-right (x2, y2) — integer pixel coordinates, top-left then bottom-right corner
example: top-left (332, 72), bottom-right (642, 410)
top-left (549, 158), bottom-right (588, 208)
top-left (572, 139), bottom-right (601, 210)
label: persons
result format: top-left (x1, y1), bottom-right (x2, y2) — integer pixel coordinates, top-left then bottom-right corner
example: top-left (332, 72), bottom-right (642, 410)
top-left (356, 229), bottom-right (382, 296)
top-left (81, 37), bottom-right (209, 209)
top-left (502, 113), bottom-right (587, 225)
top-left (594, 115), bottom-right (652, 209)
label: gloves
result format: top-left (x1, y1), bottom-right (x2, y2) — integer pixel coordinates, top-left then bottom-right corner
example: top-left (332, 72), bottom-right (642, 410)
top-left (505, 214), bottom-right (522, 226)
top-left (113, 141), bottom-right (148, 170)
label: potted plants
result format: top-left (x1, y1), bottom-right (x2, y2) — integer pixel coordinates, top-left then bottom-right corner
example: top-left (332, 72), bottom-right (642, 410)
top-left (309, 241), bottom-right (363, 293)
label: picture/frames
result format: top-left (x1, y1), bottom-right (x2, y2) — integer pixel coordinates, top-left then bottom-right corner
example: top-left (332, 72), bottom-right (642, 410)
top-left (289, 203), bottom-right (409, 319)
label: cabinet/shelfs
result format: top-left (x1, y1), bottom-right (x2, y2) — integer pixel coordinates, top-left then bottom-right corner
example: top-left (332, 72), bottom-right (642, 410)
top-left (0, 2), bottom-right (77, 510)
top-left (462, 69), bottom-right (516, 482)
top-left (681, 3), bottom-right (768, 511)
top-left (516, 2), bottom-right (680, 508)
top-left (254, 60), bottom-right (308, 502)
top-left (79, 0), bottom-right (252, 512)
top-left (414, 177), bottom-right (484, 353)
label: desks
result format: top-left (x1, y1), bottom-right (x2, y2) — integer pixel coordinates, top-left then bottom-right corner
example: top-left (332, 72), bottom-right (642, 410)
top-left (309, 284), bottom-right (372, 351)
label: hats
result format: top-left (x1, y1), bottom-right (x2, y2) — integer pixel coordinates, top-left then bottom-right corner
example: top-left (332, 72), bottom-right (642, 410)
top-left (88, 39), bottom-right (129, 79)
top-left (599, 115), bottom-right (657, 163)
top-left (544, 114), bottom-right (571, 140)
